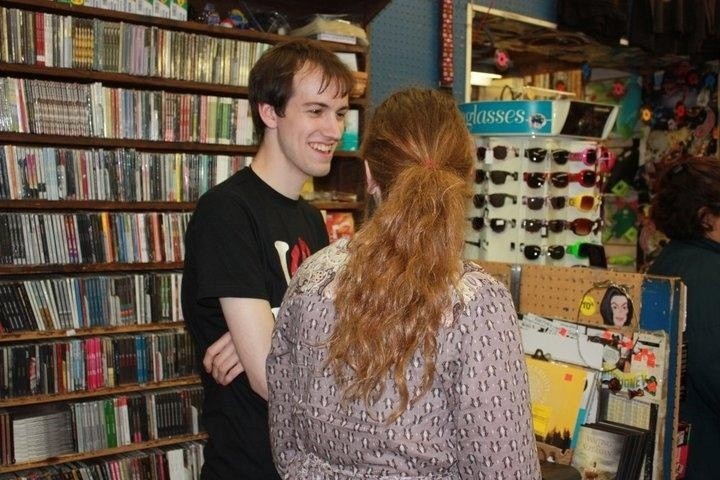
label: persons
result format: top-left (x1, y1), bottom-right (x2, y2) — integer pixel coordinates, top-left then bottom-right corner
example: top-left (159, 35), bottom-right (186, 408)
top-left (646, 156), bottom-right (720, 479)
top-left (599, 285), bottom-right (632, 329)
top-left (182, 37), bottom-right (357, 479)
top-left (265, 88), bottom-right (541, 480)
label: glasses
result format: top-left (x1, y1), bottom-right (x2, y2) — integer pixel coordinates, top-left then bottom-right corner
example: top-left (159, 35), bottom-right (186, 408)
top-left (468, 144), bottom-right (610, 260)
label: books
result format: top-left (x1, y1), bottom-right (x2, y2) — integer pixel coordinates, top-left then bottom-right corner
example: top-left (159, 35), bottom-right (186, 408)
top-left (1, 212), bottom-right (193, 265)
top-left (1, 269), bottom-right (185, 331)
top-left (517, 311), bottom-right (659, 480)
top-left (2, 383), bottom-right (207, 479)
top-left (3, 327), bottom-right (199, 400)
top-left (2, 6), bottom-right (272, 87)
top-left (2, 74), bottom-right (259, 200)
